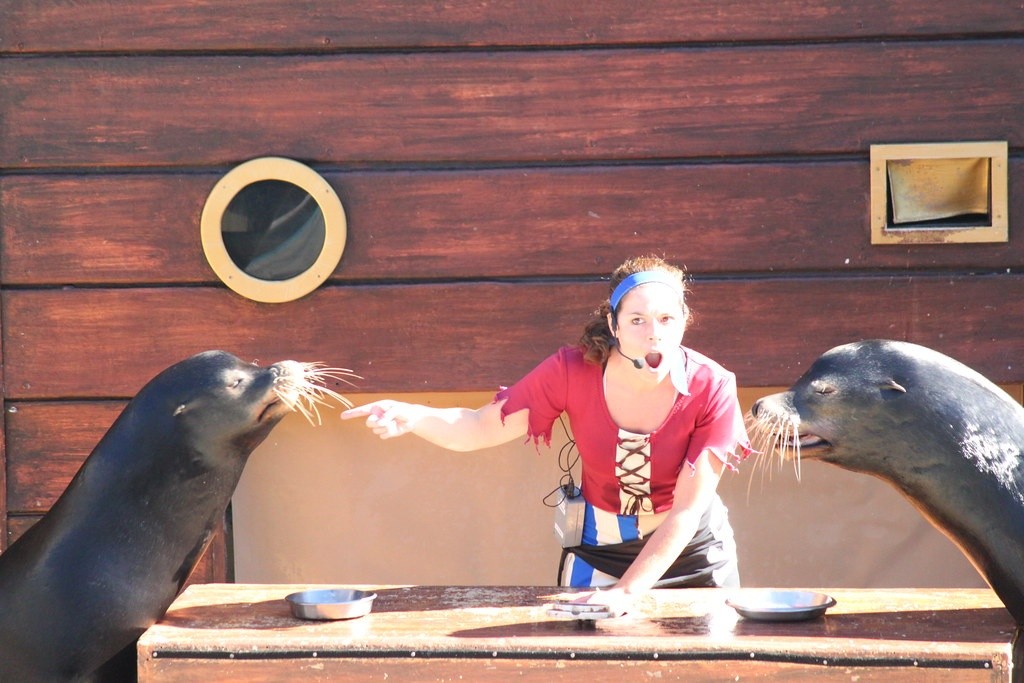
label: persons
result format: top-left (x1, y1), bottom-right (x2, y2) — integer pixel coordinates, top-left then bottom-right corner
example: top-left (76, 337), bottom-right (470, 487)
top-left (339, 254), bottom-right (751, 622)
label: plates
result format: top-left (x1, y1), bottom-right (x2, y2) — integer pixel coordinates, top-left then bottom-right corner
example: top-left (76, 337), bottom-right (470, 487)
top-left (725, 590), bottom-right (837, 620)
top-left (286, 588), bottom-right (378, 619)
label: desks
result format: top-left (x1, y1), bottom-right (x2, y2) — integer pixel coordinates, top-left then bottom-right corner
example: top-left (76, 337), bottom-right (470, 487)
top-left (135, 579), bottom-right (1024, 683)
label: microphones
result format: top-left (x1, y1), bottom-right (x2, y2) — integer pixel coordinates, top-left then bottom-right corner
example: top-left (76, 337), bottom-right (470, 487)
top-left (614, 331), bottom-right (646, 369)
